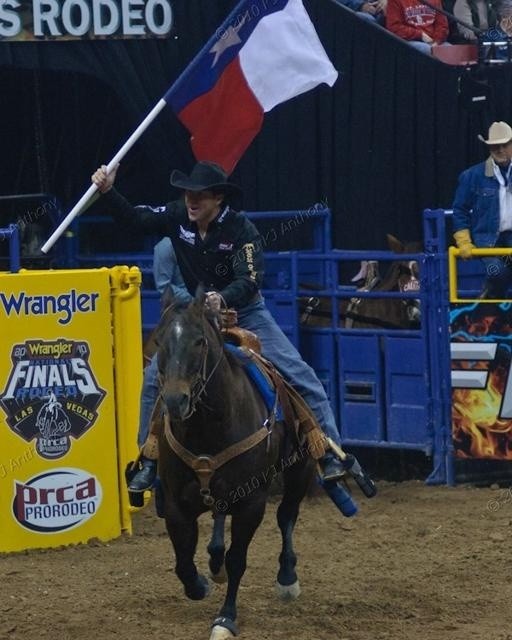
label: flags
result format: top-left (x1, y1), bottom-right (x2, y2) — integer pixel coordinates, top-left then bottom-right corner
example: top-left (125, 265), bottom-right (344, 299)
top-left (163, 1), bottom-right (341, 183)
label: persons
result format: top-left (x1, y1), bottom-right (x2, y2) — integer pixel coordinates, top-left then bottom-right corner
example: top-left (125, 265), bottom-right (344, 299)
top-left (385, 0), bottom-right (453, 57)
top-left (445, 0), bottom-right (497, 42)
top-left (452, 121), bottom-right (512, 300)
top-left (476, 2), bottom-right (512, 78)
top-left (84, 162), bottom-right (348, 493)
top-left (336, 0), bottom-right (387, 25)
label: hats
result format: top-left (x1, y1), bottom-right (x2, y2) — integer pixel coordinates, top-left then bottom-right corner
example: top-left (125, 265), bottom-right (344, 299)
top-left (170, 160), bottom-right (244, 197)
top-left (477, 121), bottom-right (512, 144)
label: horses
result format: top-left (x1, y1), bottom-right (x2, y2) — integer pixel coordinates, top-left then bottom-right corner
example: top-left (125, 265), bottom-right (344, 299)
top-left (301, 232), bottom-right (424, 482)
top-left (152, 281), bottom-right (316, 640)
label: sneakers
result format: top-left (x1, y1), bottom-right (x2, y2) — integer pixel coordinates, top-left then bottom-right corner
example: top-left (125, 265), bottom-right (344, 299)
top-left (318, 457), bottom-right (345, 482)
top-left (127, 458), bottom-right (157, 493)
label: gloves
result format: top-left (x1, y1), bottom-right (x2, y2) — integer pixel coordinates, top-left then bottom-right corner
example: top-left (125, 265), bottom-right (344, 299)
top-left (454, 229), bottom-right (476, 260)
